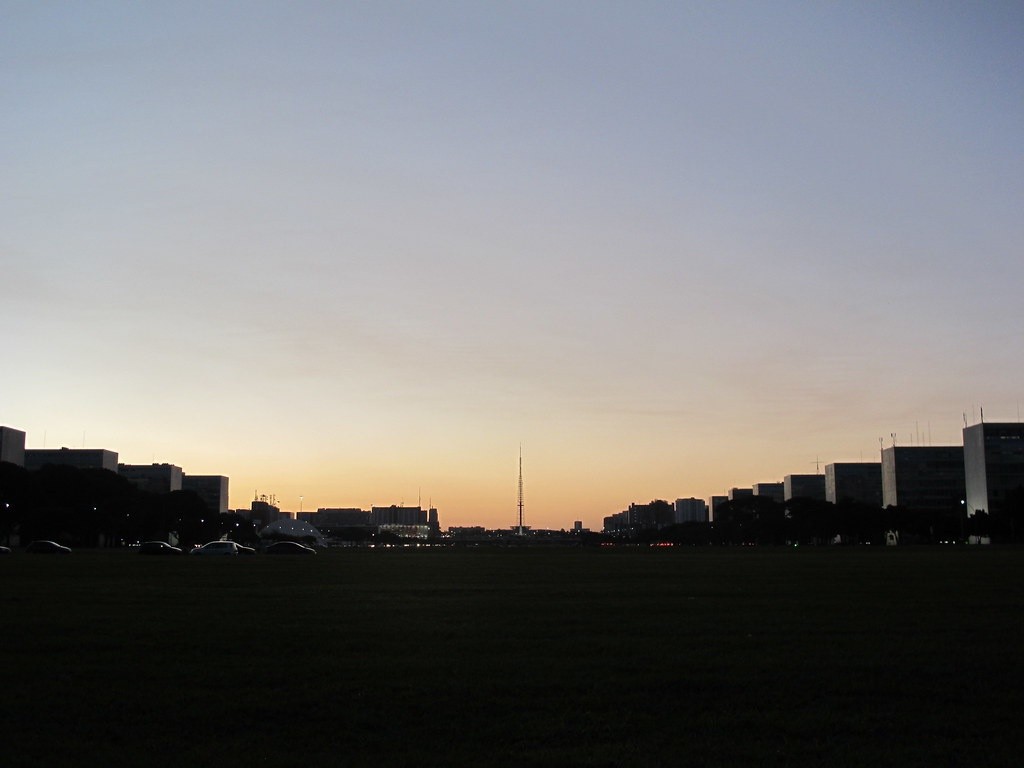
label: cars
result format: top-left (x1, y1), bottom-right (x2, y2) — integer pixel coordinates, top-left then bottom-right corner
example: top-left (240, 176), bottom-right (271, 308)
top-left (191, 541), bottom-right (239, 557)
top-left (234, 541), bottom-right (257, 555)
top-left (265, 541), bottom-right (318, 556)
top-left (0, 546), bottom-right (12, 554)
top-left (139, 542), bottom-right (183, 555)
top-left (23, 540), bottom-right (72, 554)
